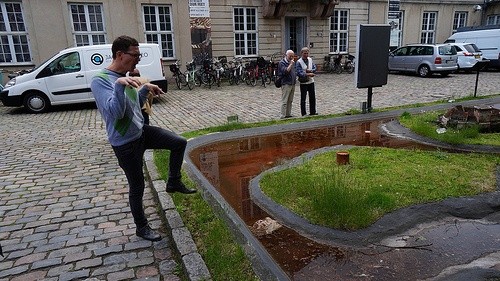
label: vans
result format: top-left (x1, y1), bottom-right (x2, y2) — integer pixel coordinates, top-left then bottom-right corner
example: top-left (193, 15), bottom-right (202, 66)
top-left (443, 29), bottom-right (500, 68)
top-left (0, 42), bottom-right (168, 114)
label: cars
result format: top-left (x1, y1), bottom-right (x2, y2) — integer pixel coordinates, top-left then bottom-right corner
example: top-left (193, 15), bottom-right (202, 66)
top-left (387, 43), bottom-right (459, 78)
top-left (446, 42), bottom-right (485, 72)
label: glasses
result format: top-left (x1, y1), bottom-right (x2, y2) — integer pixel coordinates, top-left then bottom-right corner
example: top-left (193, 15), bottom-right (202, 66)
top-left (124, 53), bottom-right (142, 59)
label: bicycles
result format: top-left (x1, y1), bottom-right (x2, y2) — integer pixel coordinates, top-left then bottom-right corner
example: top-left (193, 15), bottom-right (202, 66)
top-left (168, 54), bottom-right (280, 88)
top-left (322, 52), bottom-right (355, 75)
top-left (0, 67), bottom-right (33, 93)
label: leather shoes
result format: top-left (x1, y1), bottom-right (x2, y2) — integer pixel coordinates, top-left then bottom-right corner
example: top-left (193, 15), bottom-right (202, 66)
top-left (166, 182), bottom-right (197, 193)
top-left (135, 223), bottom-right (162, 241)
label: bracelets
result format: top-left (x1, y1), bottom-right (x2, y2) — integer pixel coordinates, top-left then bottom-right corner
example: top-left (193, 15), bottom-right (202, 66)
top-left (290, 64), bottom-right (293, 67)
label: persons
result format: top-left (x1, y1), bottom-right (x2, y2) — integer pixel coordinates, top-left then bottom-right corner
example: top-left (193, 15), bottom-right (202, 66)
top-left (278, 47), bottom-right (319, 119)
top-left (90, 35), bottom-right (198, 242)
